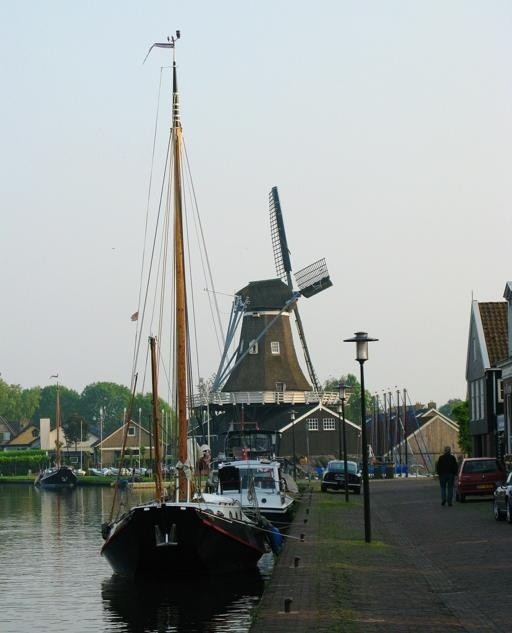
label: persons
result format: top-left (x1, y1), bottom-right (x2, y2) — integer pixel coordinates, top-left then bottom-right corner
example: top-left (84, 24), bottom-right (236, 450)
top-left (435, 445), bottom-right (458, 506)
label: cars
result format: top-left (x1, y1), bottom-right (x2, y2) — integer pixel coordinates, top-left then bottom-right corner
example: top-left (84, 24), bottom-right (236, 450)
top-left (454, 457), bottom-right (512, 522)
top-left (321, 460), bottom-right (362, 494)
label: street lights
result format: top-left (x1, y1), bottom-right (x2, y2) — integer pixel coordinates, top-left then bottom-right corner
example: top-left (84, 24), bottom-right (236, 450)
top-left (333, 331), bottom-right (379, 541)
top-left (288, 409), bottom-right (300, 457)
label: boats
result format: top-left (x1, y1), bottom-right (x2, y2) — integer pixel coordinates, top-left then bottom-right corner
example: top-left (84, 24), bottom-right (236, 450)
top-left (198, 458), bottom-right (299, 514)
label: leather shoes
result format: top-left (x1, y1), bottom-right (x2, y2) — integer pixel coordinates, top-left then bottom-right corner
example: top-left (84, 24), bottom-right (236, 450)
top-left (441, 500), bottom-right (453, 507)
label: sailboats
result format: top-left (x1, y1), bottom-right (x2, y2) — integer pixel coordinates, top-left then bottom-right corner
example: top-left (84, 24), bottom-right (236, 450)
top-left (36, 382), bottom-right (79, 488)
top-left (101, 29), bottom-right (268, 585)
